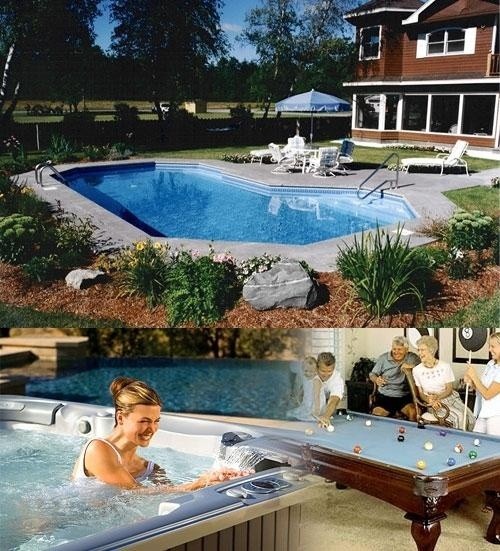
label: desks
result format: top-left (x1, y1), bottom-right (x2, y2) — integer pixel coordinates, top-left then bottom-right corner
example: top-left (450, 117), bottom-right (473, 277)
top-left (263, 410), bottom-right (500, 550)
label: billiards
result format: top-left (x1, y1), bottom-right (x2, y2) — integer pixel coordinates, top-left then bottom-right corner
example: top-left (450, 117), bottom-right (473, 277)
top-left (306, 414), bottom-right (481, 469)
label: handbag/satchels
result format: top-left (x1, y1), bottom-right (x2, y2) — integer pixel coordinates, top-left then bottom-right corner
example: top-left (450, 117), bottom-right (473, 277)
top-left (350, 356), bottom-right (374, 384)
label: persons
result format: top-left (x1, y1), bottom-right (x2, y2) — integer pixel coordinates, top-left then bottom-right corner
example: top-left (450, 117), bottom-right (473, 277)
top-left (18, 376), bottom-right (255, 541)
top-left (461, 331), bottom-right (500, 514)
top-left (287, 356), bottom-right (326, 424)
top-left (369, 336), bottom-right (421, 421)
top-left (289, 351), bottom-right (349, 429)
top-left (412, 335), bottom-right (476, 432)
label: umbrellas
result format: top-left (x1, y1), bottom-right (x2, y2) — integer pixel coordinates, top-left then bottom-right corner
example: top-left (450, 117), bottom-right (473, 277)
top-left (274, 89), bottom-right (352, 148)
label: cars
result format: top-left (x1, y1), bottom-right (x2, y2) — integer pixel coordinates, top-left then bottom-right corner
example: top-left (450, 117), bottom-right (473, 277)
top-left (30, 100), bottom-right (65, 116)
top-left (364, 95), bottom-right (389, 113)
top-left (152, 101), bottom-right (171, 113)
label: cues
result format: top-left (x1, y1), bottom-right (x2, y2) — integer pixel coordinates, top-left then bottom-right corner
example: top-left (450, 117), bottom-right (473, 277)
top-left (462, 350), bottom-right (472, 431)
top-left (295, 398), bottom-right (328, 428)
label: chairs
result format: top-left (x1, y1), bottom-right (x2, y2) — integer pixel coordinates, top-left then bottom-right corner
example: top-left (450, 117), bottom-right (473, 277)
top-left (251, 135), bottom-right (356, 179)
top-left (401, 139), bottom-right (471, 176)
top-left (401, 366), bottom-right (453, 428)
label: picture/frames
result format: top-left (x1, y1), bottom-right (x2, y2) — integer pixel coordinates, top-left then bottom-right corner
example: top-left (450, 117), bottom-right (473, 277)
top-left (403, 327), bottom-right (440, 361)
top-left (452, 327), bottom-right (496, 365)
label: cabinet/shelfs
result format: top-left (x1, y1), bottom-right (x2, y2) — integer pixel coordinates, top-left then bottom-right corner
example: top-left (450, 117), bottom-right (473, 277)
top-left (345, 379), bottom-right (475, 422)
